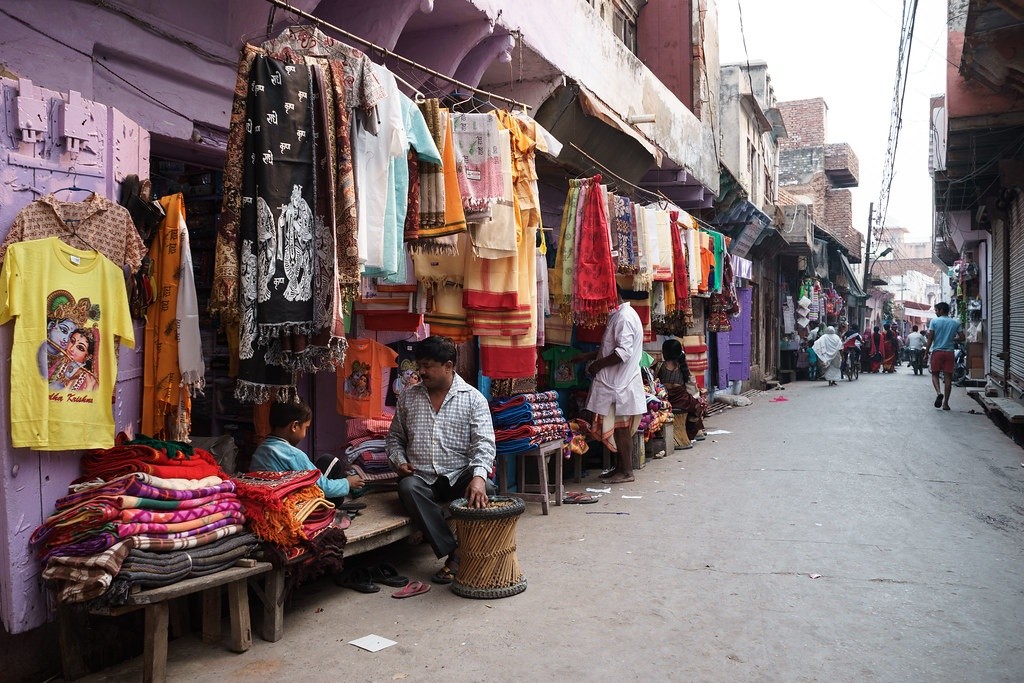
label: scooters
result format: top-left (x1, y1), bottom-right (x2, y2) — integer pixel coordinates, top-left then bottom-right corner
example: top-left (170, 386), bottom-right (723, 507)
top-left (937, 341), bottom-right (969, 386)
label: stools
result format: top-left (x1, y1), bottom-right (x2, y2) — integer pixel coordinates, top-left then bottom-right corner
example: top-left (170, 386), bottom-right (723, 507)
top-left (494, 440), bottom-right (572, 515)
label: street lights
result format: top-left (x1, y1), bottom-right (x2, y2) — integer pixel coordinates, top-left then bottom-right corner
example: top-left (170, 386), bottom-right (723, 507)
top-left (860, 247), bottom-right (894, 335)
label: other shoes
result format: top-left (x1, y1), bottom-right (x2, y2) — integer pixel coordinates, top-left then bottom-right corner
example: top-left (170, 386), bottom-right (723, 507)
top-left (692, 428), bottom-right (706, 440)
top-left (935, 394), bottom-right (944, 407)
top-left (943, 405), bottom-right (950, 410)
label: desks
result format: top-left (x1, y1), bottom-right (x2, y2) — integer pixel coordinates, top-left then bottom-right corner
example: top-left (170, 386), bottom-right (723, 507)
top-left (60, 556), bottom-right (274, 683)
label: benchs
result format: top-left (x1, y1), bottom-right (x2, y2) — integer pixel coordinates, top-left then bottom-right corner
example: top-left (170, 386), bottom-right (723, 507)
top-left (252, 490), bottom-right (414, 640)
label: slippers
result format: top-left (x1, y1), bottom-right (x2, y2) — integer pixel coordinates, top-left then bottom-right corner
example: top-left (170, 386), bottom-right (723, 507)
top-left (565, 492), bottom-right (598, 504)
top-left (335, 495), bottom-right (367, 514)
top-left (330, 563), bottom-right (380, 592)
top-left (391, 581), bottom-right (431, 599)
top-left (357, 561), bottom-right (409, 587)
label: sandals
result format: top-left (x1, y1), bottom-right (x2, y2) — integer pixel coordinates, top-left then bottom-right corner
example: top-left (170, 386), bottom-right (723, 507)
top-left (431, 558), bottom-right (460, 583)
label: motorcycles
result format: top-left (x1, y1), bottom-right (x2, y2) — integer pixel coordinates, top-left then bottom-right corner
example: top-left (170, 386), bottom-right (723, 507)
top-left (908, 344), bottom-right (925, 375)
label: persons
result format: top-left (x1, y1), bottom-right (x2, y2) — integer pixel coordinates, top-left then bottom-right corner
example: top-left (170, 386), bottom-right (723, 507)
top-left (812, 326), bottom-right (844, 385)
top-left (586, 282), bottom-right (648, 482)
top-left (385, 335), bottom-right (496, 582)
top-left (806, 321), bottom-right (819, 342)
top-left (806, 339), bottom-right (817, 381)
top-left (841, 324), bottom-right (865, 373)
top-left (924, 302), bottom-right (965, 410)
top-left (871, 324), bottom-right (900, 373)
top-left (905, 325), bottom-right (926, 367)
top-left (250, 396), bottom-right (365, 532)
top-left (650, 339), bottom-right (708, 442)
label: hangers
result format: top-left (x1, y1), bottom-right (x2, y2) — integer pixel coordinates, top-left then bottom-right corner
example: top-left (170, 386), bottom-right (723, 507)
top-left (48, 169), bottom-right (100, 202)
top-left (57, 216), bottom-right (98, 253)
top-left (348, 317), bottom-right (375, 347)
top-left (568, 155), bottom-right (722, 228)
top-left (234, 0), bottom-right (544, 119)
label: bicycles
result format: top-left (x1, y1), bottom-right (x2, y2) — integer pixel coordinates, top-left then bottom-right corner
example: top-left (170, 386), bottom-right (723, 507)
top-left (845, 344), bottom-right (864, 381)
